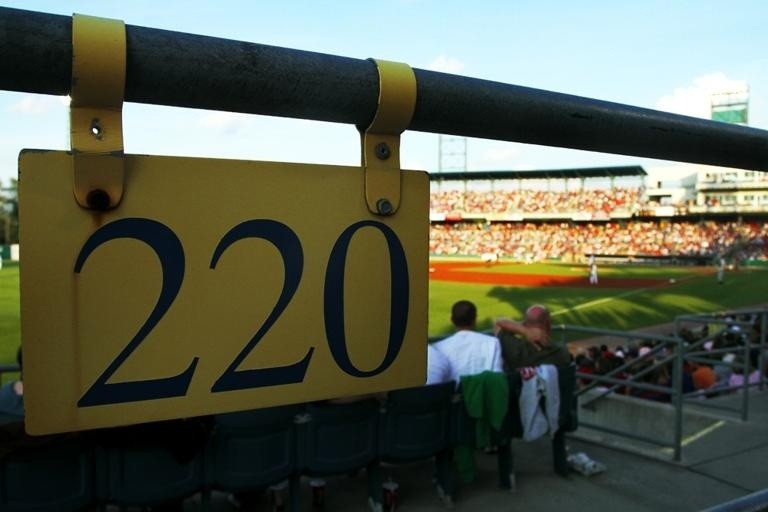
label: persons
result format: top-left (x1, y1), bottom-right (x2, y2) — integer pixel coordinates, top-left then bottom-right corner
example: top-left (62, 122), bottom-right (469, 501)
top-left (491, 302), bottom-right (578, 435)
top-left (1, 345), bottom-right (23, 420)
top-left (587, 253), bottom-right (599, 286)
top-left (427, 187), bottom-right (768, 267)
top-left (571, 308), bottom-right (767, 406)
top-left (423, 342), bottom-right (452, 385)
top-left (433, 298), bottom-right (505, 388)
top-left (714, 255), bottom-right (726, 284)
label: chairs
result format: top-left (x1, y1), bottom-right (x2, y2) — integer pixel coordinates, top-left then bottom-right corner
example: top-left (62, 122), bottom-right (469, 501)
top-left (0, 363), bottom-right (581, 512)
